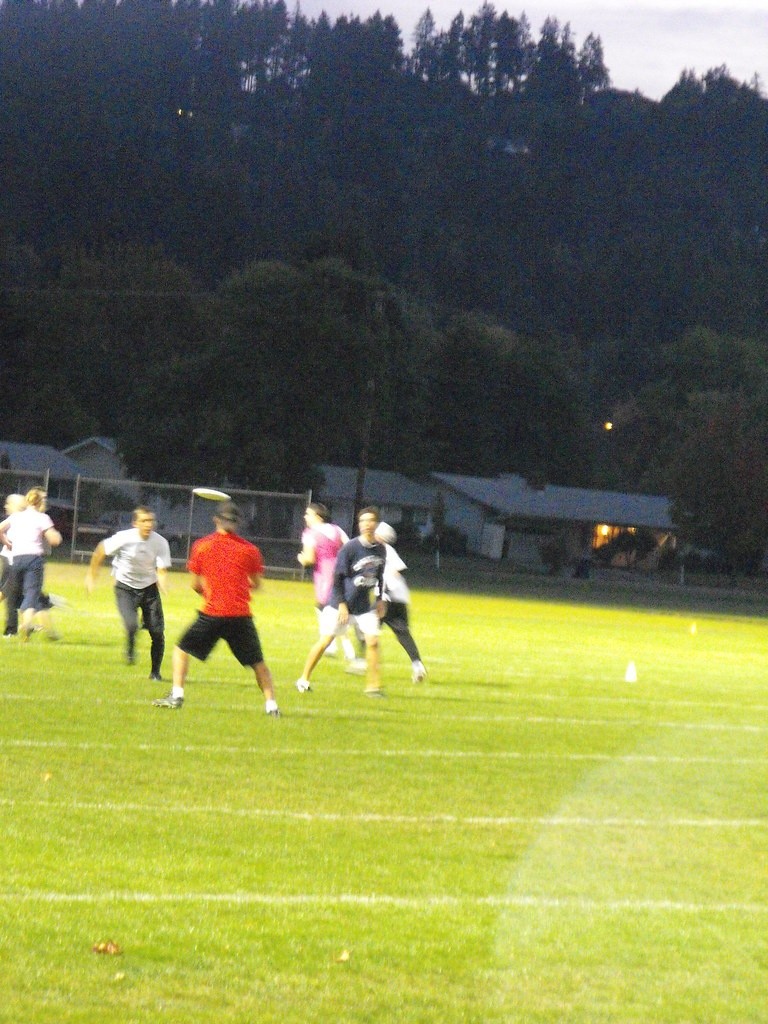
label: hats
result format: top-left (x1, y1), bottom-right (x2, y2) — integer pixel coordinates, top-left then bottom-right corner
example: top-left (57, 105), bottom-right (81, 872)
top-left (374, 521), bottom-right (397, 545)
top-left (215, 501), bottom-right (239, 521)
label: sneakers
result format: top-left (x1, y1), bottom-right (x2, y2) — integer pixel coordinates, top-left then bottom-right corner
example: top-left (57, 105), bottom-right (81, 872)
top-left (152, 695), bottom-right (184, 709)
top-left (267, 709), bottom-right (282, 718)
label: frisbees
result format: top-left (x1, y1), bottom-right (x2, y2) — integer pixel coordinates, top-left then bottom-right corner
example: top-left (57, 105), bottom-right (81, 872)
top-left (192, 488), bottom-right (230, 500)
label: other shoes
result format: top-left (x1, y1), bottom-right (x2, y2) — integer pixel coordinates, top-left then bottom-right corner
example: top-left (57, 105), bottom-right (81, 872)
top-left (2, 626), bottom-right (18, 637)
top-left (364, 688), bottom-right (383, 700)
top-left (149, 673), bottom-right (161, 680)
top-left (411, 661), bottom-right (426, 683)
top-left (350, 660), bottom-right (368, 672)
top-left (127, 657), bottom-right (134, 665)
top-left (297, 679), bottom-right (312, 693)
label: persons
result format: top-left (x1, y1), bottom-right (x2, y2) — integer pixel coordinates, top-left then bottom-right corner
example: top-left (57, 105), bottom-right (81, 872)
top-left (84, 504), bottom-right (173, 682)
top-left (0, 486), bottom-right (71, 642)
top-left (296, 506), bottom-right (385, 698)
top-left (297, 502), bottom-right (355, 659)
top-left (152, 502), bottom-right (278, 716)
top-left (344, 521), bottom-right (426, 683)
